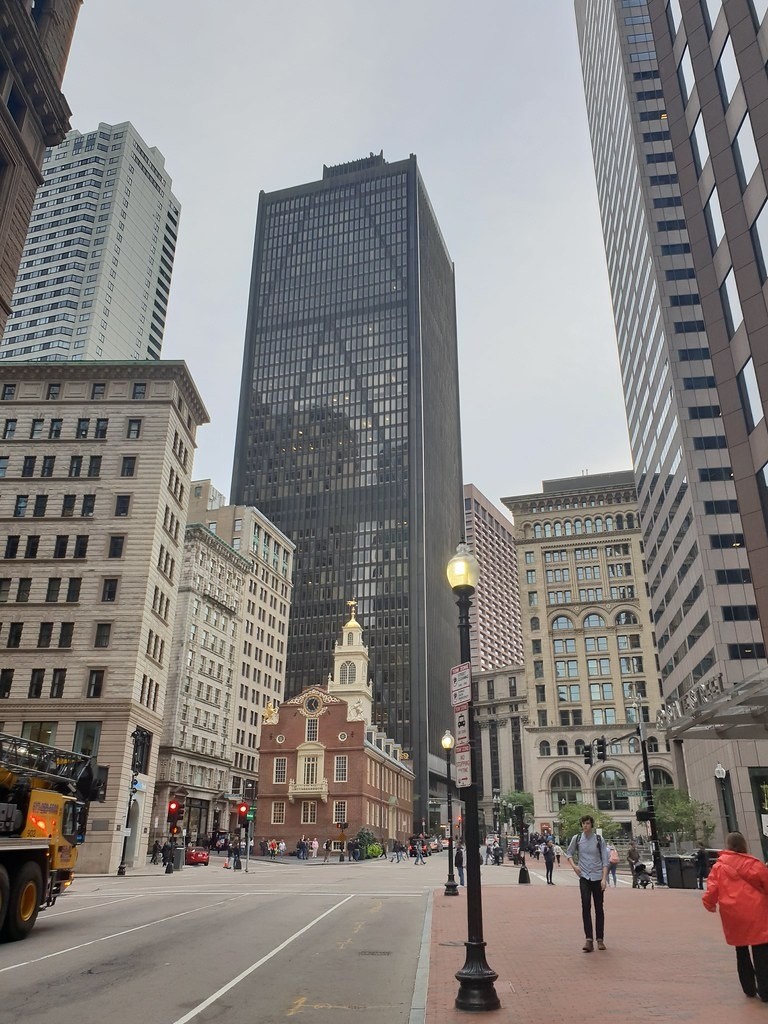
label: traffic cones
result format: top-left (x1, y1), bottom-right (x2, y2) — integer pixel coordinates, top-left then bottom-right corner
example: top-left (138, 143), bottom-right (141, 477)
top-left (222, 857), bottom-right (228, 868)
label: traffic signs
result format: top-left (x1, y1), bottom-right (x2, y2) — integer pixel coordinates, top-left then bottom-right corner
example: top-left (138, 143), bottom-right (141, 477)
top-left (451, 702), bottom-right (470, 747)
top-left (453, 744), bottom-right (473, 788)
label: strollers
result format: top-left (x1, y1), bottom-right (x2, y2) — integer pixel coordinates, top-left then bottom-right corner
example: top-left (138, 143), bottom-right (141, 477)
top-left (631, 859), bottom-right (655, 890)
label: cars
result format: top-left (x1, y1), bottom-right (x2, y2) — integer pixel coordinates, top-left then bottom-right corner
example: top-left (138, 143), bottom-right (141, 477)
top-left (173, 846), bottom-right (209, 867)
top-left (485, 834), bottom-right (499, 845)
top-left (409, 837), bottom-right (449, 856)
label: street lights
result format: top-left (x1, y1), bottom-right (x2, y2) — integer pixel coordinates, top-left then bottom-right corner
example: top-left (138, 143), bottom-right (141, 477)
top-left (211, 807), bottom-right (221, 850)
top-left (116, 728), bottom-right (150, 875)
top-left (493, 794), bottom-right (517, 831)
top-left (441, 538), bottom-right (504, 1012)
top-left (441, 729), bottom-right (459, 896)
top-left (714, 761), bottom-right (732, 834)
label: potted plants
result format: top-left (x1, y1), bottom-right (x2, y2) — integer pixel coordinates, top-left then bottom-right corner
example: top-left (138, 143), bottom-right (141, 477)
top-left (651, 786), bottom-right (715, 889)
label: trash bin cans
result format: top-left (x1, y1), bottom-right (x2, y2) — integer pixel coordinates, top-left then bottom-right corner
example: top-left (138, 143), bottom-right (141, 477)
top-left (663, 855), bottom-right (699, 890)
top-left (353, 842), bottom-right (365, 860)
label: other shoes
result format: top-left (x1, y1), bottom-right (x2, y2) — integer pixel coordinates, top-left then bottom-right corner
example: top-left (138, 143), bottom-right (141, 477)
top-left (415, 863), bottom-right (418, 865)
top-left (550, 882), bottom-right (555, 885)
top-left (547, 881), bottom-right (550, 884)
top-left (424, 862), bottom-right (426, 864)
top-left (457, 885), bottom-right (464, 887)
top-left (762, 997), bottom-right (768, 1001)
top-left (751, 990), bottom-right (756, 996)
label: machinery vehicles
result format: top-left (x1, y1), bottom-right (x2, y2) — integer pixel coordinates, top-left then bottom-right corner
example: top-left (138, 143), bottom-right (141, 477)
top-left (0, 728), bottom-right (110, 944)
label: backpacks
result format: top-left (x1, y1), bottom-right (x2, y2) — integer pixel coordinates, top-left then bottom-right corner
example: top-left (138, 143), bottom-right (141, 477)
top-left (608, 847), bottom-right (619, 863)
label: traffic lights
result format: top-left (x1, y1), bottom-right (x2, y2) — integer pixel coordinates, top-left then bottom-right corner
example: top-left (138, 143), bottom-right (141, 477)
top-left (343, 823), bottom-right (348, 828)
top-left (170, 825), bottom-right (178, 835)
top-left (336, 823), bottom-right (342, 828)
top-left (166, 800), bottom-right (179, 822)
top-left (583, 742), bottom-right (593, 767)
top-left (239, 803), bottom-right (248, 815)
top-left (177, 803), bottom-right (184, 821)
top-left (596, 737), bottom-right (608, 761)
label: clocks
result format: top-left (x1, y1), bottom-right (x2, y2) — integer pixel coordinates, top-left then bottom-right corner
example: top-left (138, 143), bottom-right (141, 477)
top-left (306, 697), bottom-right (319, 712)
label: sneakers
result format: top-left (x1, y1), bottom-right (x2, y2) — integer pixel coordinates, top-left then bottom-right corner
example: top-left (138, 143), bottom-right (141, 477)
top-left (597, 940), bottom-right (606, 950)
top-left (582, 940), bottom-right (594, 952)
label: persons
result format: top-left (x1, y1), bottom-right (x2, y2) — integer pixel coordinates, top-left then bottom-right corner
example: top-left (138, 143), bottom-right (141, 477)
top-left (259, 837), bottom-right (267, 856)
top-left (347, 837), bottom-right (362, 861)
top-left (324, 839), bottom-right (332, 862)
top-left (279, 839), bottom-right (286, 860)
top-left (380, 844), bottom-right (387, 858)
top-left (606, 842), bottom-right (619, 887)
top-left (240, 838), bottom-right (254, 855)
top-left (390, 839), bottom-right (427, 865)
top-left (627, 841), bottom-right (640, 887)
top-left (543, 839), bottom-right (555, 885)
top-left (485, 841), bottom-right (500, 866)
top-left (216, 839), bottom-right (221, 854)
top-left (703, 831), bottom-right (768, 1002)
top-left (269, 838), bottom-right (277, 860)
top-left (528, 832), bottom-right (561, 867)
top-left (150, 840), bottom-right (171, 865)
top-left (296, 837), bottom-right (319, 858)
top-left (455, 843), bottom-right (464, 885)
top-left (566, 815), bottom-right (609, 950)
top-left (228, 844), bottom-right (240, 871)
